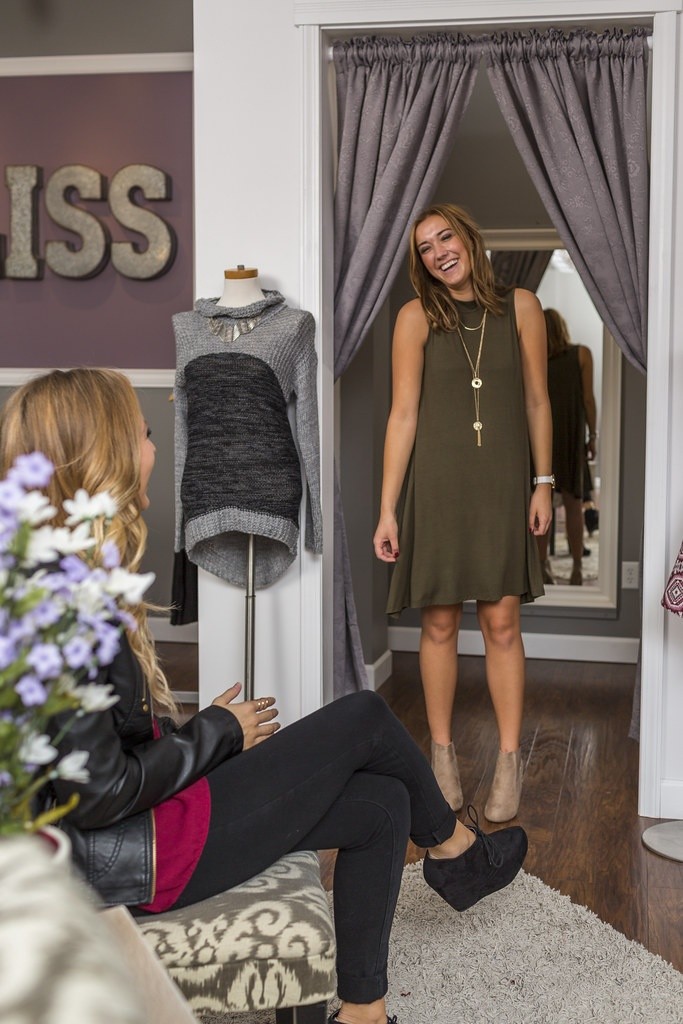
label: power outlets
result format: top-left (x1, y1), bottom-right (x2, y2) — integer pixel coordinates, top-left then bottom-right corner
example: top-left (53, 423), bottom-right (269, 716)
top-left (622, 561), bottom-right (639, 589)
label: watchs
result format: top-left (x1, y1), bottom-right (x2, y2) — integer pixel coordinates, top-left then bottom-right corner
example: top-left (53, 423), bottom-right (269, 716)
top-left (589, 433), bottom-right (598, 438)
top-left (532, 474), bottom-right (555, 489)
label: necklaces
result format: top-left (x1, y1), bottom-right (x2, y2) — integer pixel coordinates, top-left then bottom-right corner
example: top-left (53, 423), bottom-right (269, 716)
top-left (447, 294), bottom-right (488, 447)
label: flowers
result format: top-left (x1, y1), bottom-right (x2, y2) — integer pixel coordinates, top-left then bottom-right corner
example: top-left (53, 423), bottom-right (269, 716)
top-left (0, 454), bottom-right (158, 869)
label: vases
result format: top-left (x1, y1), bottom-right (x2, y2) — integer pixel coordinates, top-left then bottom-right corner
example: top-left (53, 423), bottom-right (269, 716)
top-left (0, 821), bottom-right (136, 1024)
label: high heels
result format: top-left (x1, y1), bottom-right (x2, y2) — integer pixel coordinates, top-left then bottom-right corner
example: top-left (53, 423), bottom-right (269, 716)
top-left (584, 506), bottom-right (599, 537)
top-left (542, 569), bottom-right (554, 584)
top-left (483, 743), bottom-right (524, 823)
top-left (569, 571), bottom-right (583, 585)
top-left (429, 737), bottom-right (464, 812)
top-left (424, 805), bottom-right (528, 912)
top-left (326, 1007), bottom-right (398, 1024)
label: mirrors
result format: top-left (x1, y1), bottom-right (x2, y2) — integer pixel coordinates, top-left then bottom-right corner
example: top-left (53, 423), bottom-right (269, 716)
top-left (463, 229), bottom-right (621, 609)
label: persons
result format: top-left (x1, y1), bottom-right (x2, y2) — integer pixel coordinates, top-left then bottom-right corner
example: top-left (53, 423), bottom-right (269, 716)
top-left (0, 367), bottom-right (529, 1024)
top-left (533, 309), bottom-right (598, 585)
top-left (371, 204), bottom-right (556, 824)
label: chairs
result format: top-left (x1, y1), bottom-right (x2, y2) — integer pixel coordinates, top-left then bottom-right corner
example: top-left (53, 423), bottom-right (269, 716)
top-left (132, 845), bottom-right (340, 1024)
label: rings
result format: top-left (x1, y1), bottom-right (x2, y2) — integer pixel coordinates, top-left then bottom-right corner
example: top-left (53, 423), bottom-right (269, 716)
top-left (253, 697), bottom-right (269, 711)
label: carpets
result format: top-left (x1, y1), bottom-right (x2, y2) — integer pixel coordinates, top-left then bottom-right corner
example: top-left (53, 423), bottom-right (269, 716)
top-left (206, 857), bottom-right (683, 1024)
